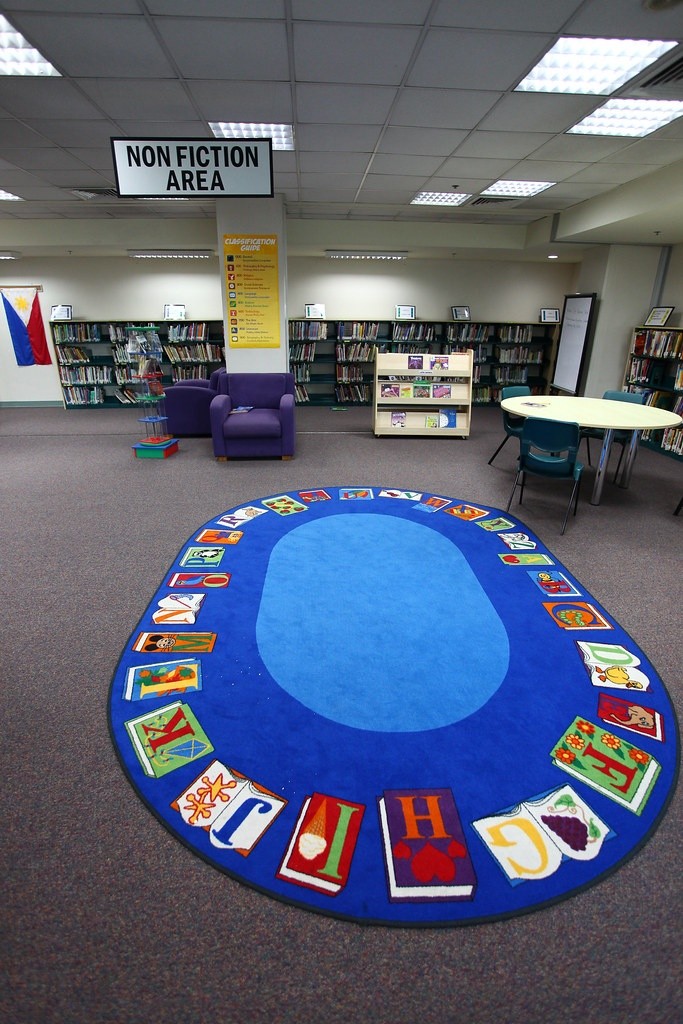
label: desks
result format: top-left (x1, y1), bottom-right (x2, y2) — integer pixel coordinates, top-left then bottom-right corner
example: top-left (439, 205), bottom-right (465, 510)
top-left (501, 395), bottom-right (683, 506)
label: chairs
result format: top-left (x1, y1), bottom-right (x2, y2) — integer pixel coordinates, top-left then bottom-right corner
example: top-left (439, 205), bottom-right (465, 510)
top-left (576, 390), bottom-right (642, 484)
top-left (505, 417), bottom-right (584, 537)
top-left (488, 386), bottom-right (554, 465)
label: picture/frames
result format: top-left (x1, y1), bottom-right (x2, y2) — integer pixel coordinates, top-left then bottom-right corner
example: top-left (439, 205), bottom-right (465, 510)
top-left (540, 308), bottom-right (560, 323)
top-left (51, 304), bottom-right (72, 321)
top-left (643, 306), bottom-right (675, 327)
top-left (164, 304), bottom-right (186, 320)
top-left (451, 305), bottom-right (471, 321)
top-left (395, 305), bottom-right (416, 320)
top-left (305, 304), bottom-right (325, 319)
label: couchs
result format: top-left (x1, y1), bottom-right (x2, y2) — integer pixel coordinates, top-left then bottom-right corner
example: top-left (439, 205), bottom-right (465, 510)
top-left (210, 372), bottom-right (295, 461)
top-left (159, 367), bottom-right (225, 438)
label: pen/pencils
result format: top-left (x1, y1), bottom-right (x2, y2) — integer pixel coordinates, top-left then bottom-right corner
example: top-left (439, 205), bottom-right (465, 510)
top-left (524, 402), bottom-right (550, 407)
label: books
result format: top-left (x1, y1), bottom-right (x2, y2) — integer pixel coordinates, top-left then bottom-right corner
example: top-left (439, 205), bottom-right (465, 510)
top-left (393, 322), bottom-right (436, 353)
top-left (288, 321), bottom-right (328, 403)
top-left (229, 406), bottom-right (253, 414)
top-left (623, 330), bottom-right (683, 455)
top-left (108, 323), bottom-right (164, 403)
top-left (163, 323), bottom-right (225, 382)
top-left (330, 406), bottom-right (348, 411)
top-left (381, 356), bottom-right (456, 428)
top-left (493, 325), bottom-right (544, 402)
top-left (442, 324), bottom-right (492, 403)
top-left (53, 324), bottom-right (113, 405)
top-left (336, 322), bottom-right (390, 402)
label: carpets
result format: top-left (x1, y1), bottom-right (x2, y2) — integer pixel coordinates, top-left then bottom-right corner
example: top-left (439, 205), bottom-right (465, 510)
top-left (106, 486), bottom-right (682, 927)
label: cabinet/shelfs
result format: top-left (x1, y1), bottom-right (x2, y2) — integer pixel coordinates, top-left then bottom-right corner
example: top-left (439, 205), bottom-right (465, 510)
top-left (621, 326), bottom-right (683, 463)
top-left (50, 318), bottom-right (557, 438)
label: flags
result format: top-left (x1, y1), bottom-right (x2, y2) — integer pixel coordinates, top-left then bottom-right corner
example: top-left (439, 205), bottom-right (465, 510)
top-left (1, 289), bottom-right (52, 366)
